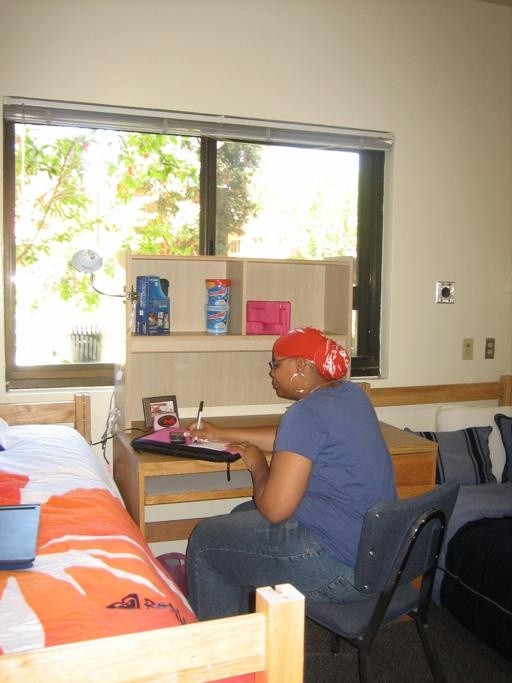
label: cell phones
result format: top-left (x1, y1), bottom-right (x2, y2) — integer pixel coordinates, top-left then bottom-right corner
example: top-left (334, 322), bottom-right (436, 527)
top-left (169, 432), bottom-right (186, 443)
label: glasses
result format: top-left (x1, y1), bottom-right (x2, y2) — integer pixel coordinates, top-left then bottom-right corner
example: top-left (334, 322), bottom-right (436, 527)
top-left (267, 358), bottom-right (285, 368)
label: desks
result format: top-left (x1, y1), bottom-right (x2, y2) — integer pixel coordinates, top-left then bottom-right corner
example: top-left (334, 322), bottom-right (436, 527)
top-left (110, 413), bottom-right (444, 624)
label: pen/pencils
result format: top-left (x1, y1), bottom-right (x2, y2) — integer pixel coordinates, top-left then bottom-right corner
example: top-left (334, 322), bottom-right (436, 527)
top-left (194, 401), bottom-right (203, 445)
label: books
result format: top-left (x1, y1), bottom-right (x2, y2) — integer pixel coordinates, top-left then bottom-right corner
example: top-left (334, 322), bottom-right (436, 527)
top-left (0, 505), bottom-right (41, 571)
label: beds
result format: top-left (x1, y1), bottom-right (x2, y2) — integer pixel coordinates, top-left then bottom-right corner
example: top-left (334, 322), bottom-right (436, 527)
top-left (358, 374), bottom-right (512, 667)
top-left (0, 389), bottom-right (304, 681)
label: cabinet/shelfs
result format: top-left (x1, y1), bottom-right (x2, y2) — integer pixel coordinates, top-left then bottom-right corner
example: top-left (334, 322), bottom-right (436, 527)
top-left (123, 248), bottom-right (354, 435)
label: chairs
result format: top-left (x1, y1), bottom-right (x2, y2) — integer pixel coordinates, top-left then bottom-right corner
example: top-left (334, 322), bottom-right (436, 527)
top-left (308, 482), bottom-right (461, 683)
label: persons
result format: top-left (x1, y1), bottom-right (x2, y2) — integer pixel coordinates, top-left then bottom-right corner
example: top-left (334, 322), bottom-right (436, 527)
top-left (187, 326), bottom-right (406, 620)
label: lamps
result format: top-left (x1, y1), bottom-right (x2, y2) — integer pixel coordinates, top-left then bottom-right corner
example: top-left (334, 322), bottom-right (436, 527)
top-left (69, 249), bottom-right (137, 304)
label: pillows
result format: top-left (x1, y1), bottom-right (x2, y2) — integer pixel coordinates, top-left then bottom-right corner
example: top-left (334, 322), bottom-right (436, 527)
top-left (436, 402), bottom-right (512, 483)
top-left (494, 413), bottom-right (512, 483)
top-left (402, 426), bottom-right (497, 490)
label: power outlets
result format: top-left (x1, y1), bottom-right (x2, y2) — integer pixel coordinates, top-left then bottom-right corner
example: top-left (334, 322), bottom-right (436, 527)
top-left (485, 337), bottom-right (495, 359)
top-left (462, 338), bottom-right (474, 360)
